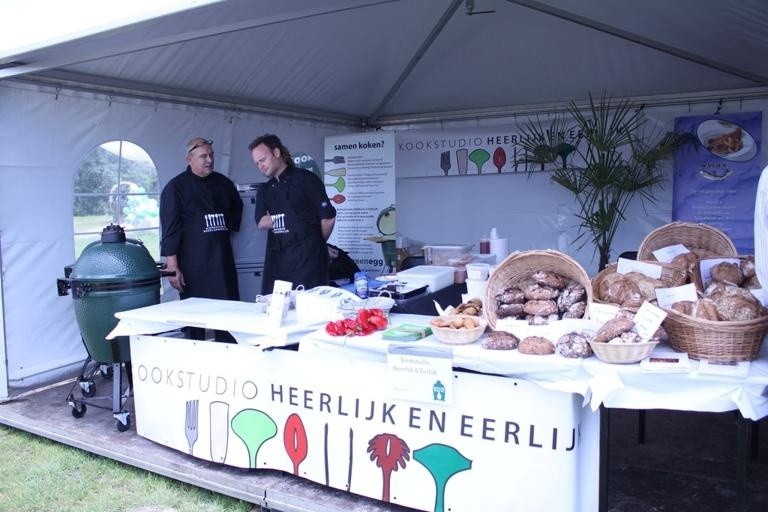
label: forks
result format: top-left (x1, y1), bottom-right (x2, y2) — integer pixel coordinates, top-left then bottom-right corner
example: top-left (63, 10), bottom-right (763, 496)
top-left (440, 151), bottom-right (451, 176)
top-left (323, 156), bottom-right (345, 164)
top-left (184, 399), bottom-right (199, 456)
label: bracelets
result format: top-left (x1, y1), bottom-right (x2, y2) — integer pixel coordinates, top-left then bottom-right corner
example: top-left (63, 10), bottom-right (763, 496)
top-left (464, 262), bottom-right (489, 280)
top-left (464, 279), bottom-right (489, 295)
top-left (489, 264), bottom-right (499, 280)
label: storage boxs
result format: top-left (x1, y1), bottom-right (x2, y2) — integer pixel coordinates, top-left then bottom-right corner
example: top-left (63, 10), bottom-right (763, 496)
top-left (397, 243), bottom-right (497, 293)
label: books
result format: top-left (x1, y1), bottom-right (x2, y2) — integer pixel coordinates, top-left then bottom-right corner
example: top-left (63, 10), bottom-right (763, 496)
top-left (397, 243), bottom-right (497, 293)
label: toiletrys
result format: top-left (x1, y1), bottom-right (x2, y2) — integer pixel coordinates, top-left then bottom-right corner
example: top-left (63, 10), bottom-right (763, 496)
top-left (325, 309), bottom-right (388, 337)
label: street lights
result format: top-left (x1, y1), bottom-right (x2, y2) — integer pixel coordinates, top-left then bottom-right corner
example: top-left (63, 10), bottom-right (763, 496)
top-left (326, 243), bottom-right (361, 287)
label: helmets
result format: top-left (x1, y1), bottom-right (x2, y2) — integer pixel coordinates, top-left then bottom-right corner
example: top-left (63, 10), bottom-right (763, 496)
top-left (698, 127), bottom-right (753, 160)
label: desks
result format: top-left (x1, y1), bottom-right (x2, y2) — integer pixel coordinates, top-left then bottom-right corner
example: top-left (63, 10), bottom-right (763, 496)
top-left (130, 279), bottom-right (767, 512)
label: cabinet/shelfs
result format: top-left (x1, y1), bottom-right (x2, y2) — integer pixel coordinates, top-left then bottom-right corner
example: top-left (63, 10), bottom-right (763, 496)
top-left (479, 238), bottom-right (490, 254)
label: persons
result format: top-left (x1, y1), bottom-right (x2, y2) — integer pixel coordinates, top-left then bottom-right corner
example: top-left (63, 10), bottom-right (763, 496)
top-left (247, 130), bottom-right (336, 353)
top-left (158, 137), bottom-right (245, 343)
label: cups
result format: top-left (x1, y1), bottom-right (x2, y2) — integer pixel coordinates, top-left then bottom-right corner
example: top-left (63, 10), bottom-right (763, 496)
top-left (188, 140), bottom-right (213, 153)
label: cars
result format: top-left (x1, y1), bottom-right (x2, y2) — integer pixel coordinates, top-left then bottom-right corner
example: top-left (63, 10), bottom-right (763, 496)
top-left (354, 271), bottom-right (369, 299)
